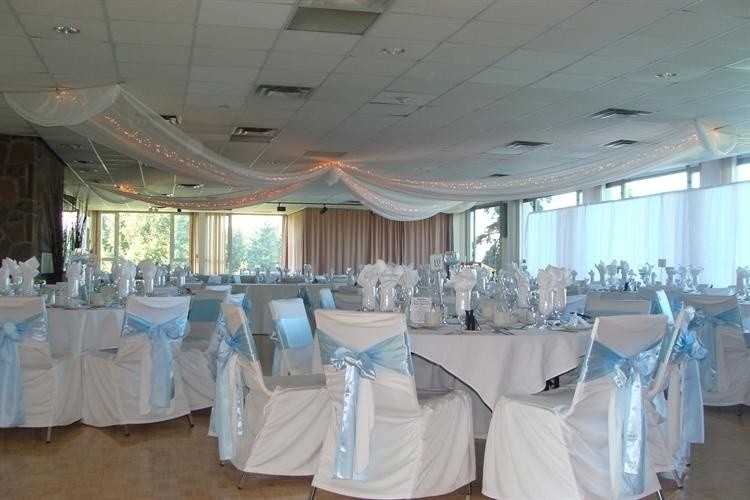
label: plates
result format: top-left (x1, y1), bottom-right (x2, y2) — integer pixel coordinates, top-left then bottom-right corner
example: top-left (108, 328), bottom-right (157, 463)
top-left (422, 324), bottom-right (445, 329)
top-left (489, 322), bottom-right (513, 329)
top-left (422, 324), bottom-right (449, 328)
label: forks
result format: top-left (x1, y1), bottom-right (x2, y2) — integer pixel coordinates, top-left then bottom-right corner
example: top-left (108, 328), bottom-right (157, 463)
top-left (494, 328), bottom-right (515, 335)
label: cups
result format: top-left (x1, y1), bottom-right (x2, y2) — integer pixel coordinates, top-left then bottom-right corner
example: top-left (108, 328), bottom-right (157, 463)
top-left (482, 306), bottom-right (495, 320)
top-left (481, 306), bottom-right (497, 321)
top-left (92, 293), bottom-right (104, 307)
top-left (425, 312), bottom-right (444, 324)
top-left (493, 312), bottom-right (513, 327)
top-left (55, 297), bottom-right (69, 306)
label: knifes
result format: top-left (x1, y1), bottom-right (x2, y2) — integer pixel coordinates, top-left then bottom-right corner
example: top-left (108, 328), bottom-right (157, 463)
top-left (557, 329), bottom-right (579, 333)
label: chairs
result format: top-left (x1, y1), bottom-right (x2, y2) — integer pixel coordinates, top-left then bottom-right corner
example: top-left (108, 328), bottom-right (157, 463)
top-left (178, 293), bottom-right (247, 411)
top-left (702, 287), bottom-right (732, 295)
top-left (221, 302), bottom-right (327, 490)
top-left (696, 283), bottom-right (713, 292)
top-left (268, 298), bottom-right (314, 375)
top-left (1, 295), bottom-right (83, 444)
top-left (565, 305), bottom-right (696, 490)
top-left (655, 289), bottom-right (677, 344)
top-left (500, 314), bottom-right (668, 499)
top-left (318, 288), bottom-right (337, 309)
top-left (583, 298), bottom-right (652, 322)
top-left (683, 292), bottom-right (750, 416)
top-left (310, 308), bottom-right (472, 500)
top-left (82, 294), bottom-right (194, 436)
top-left (180, 288), bottom-right (232, 352)
top-left (205, 285), bottom-right (232, 302)
top-left (638, 286), bottom-right (683, 322)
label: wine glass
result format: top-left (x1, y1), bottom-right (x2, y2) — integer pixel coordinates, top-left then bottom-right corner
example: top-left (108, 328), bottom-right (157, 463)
top-left (380, 288), bottom-right (392, 312)
top-left (24, 277), bottom-right (33, 296)
top-left (584, 279), bottom-right (590, 291)
top-left (13, 277), bottom-right (23, 296)
top-left (68, 277), bottom-right (78, 297)
top-left (680, 275), bottom-right (687, 288)
top-left (504, 288), bottom-right (520, 324)
top-left (456, 289), bottom-right (470, 328)
top-left (736, 274), bottom-right (750, 298)
top-left (527, 292), bottom-right (540, 328)
top-left (177, 276), bottom-right (185, 287)
top-left (470, 291), bottom-right (480, 315)
top-left (362, 287), bottom-right (375, 312)
top-left (129, 280), bottom-right (136, 293)
top-left (593, 268), bottom-right (658, 292)
top-left (444, 251), bottom-right (460, 271)
top-left (144, 277), bottom-right (153, 296)
top-left (552, 288), bottom-right (566, 327)
top-left (154, 275), bottom-right (165, 287)
top-left (539, 291), bottom-right (553, 330)
top-left (0, 270), bottom-right (10, 295)
top-left (400, 286), bottom-right (412, 314)
top-left (692, 273), bottom-right (699, 294)
top-left (304, 264), bottom-right (314, 283)
top-left (476, 275), bottom-right (486, 294)
top-left (119, 279), bottom-right (129, 308)
